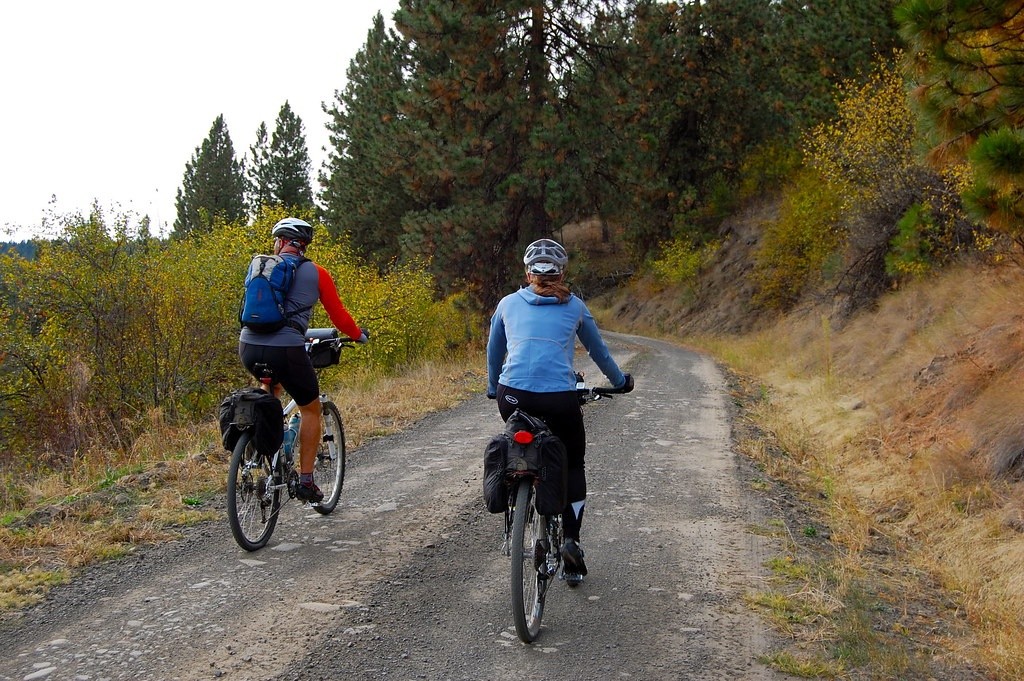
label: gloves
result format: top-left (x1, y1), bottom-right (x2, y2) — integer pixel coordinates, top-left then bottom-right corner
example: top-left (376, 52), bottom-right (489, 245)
top-left (625, 373), bottom-right (635, 391)
top-left (356, 330), bottom-right (370, 346)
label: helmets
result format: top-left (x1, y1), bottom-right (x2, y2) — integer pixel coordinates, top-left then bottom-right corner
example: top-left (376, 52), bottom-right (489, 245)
top-left (523, 238), bottom-right (569, 275)
top-left (272, 217), bottom-right (313, 244)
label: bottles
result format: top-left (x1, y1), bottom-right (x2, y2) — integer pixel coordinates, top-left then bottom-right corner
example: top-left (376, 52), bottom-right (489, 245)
top-left (282, 415), bottom-right (293, 455)
top-left (288, 413), bottom-right (300, 443)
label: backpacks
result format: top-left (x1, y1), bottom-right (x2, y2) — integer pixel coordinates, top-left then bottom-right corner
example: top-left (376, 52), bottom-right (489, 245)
top-left (242, 254), bottom-right (308, 332)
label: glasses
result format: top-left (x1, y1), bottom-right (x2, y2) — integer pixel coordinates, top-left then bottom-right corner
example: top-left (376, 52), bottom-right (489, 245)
top-left (273, 236), bottom-right (286, 243)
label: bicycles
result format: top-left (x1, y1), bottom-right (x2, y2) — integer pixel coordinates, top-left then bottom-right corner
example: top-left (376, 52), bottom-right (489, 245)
top-left (487, 373), bottom-right (634, 642)
top-left (226, 328), bottom-right (370, 553)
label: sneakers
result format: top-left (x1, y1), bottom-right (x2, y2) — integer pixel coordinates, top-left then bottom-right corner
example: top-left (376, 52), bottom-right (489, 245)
top-left (561, 538), bottom-right (588, 576)
top-left (296, 483), bottom-right (324, 502)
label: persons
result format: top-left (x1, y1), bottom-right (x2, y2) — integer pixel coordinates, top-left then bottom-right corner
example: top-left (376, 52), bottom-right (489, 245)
top-left (239, 218), bottom-right (369, 501)
top-left (486, 239), bottom-right (634, 575)
